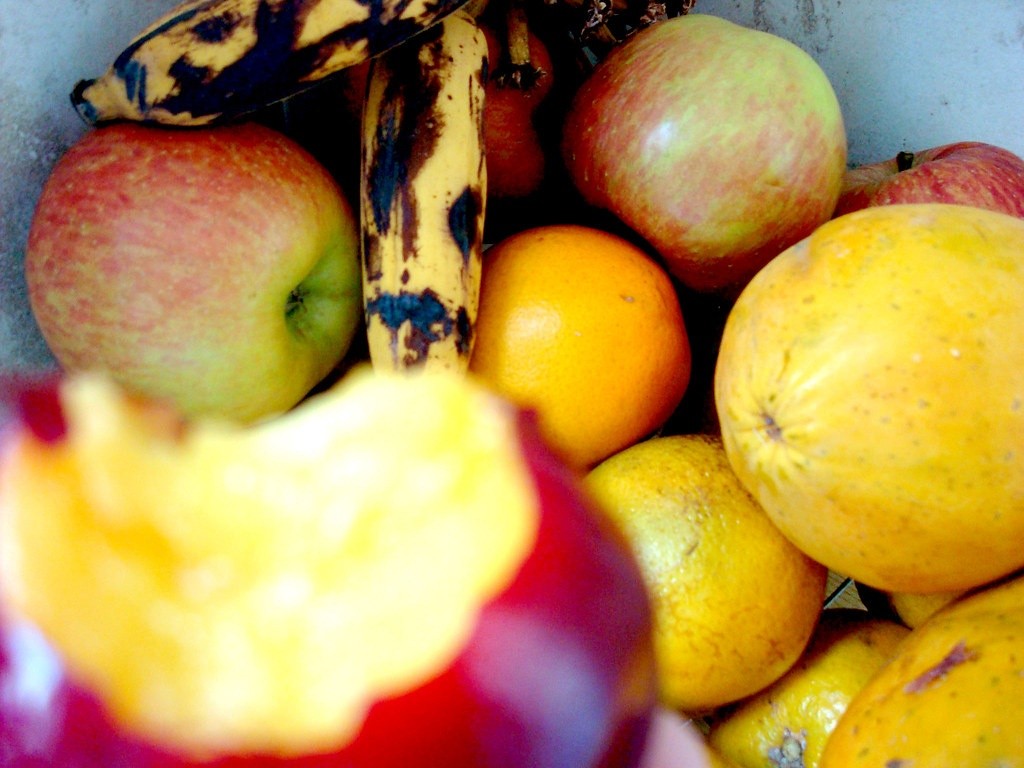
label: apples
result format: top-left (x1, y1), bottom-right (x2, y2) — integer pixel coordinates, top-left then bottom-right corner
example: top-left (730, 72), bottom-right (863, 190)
top-left (29, 12), bottom-right (1024, 425)
top-left (0, 387), bottom-right (654, 768)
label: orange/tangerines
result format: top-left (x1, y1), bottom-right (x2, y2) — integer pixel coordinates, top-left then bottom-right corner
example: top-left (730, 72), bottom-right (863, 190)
top-left (480, 204), bottom-right (1024, 768)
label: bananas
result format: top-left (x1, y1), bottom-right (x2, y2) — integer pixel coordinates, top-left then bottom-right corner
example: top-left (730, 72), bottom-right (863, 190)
top-left (70, 0), bottom-right (489, 381)
